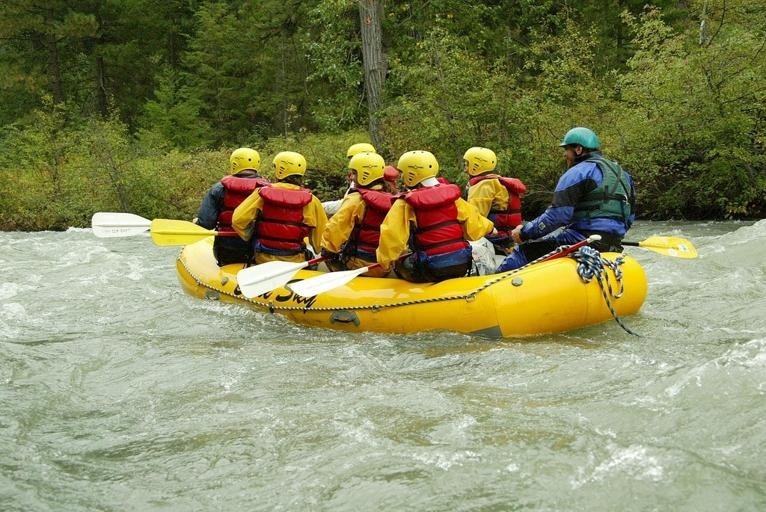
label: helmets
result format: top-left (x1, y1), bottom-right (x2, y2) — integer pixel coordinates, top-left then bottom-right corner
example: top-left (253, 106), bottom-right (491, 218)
top-left (272, 151), bottom-right (306, 180)
top-left (397, 150), bottom-right (438, 188)
top-left (229, 148), bottom-right (261, 175)
top-left (463, 146), bottom-right (497, 176)
top-left (558, 127), bottom-right (600, 152)
top-left (348, 152), bottom-right (386, 187)
top-left (347, 143), bottom-right (376, 159)
top-left (384, 164), bottom-right (399, 182)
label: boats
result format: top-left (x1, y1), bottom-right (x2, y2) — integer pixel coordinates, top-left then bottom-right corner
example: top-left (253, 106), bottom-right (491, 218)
top-left (173, 216), bottom-right (652, 340)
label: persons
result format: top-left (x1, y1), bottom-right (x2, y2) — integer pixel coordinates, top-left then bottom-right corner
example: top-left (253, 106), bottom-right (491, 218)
top-left (463, 146), bottom-right (527, 256)
top-left (192, 147), bottom-right (270, 267)
top-left (494, 127), bottom-right (637, 274)
top-left (232, 151), bottom-right (329, 270)
top-left (321, 143), bottom-right (399, 215)
top-left (375, 150), bottom-right (512, 284)
top-left (319, 151), bottom-right (403, 278)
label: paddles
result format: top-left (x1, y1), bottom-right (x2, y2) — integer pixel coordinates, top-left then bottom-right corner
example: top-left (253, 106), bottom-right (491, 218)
top-left (150, 218), bottom-right (240, 245)
top-left (92, 212), bottom-right (152, 238)
top-left (237, 253), bottom-right (337, 299)
top-left (620, 235), bottom-right (698, 259)
top-left (287, 251), bottom-right (413, 298)
